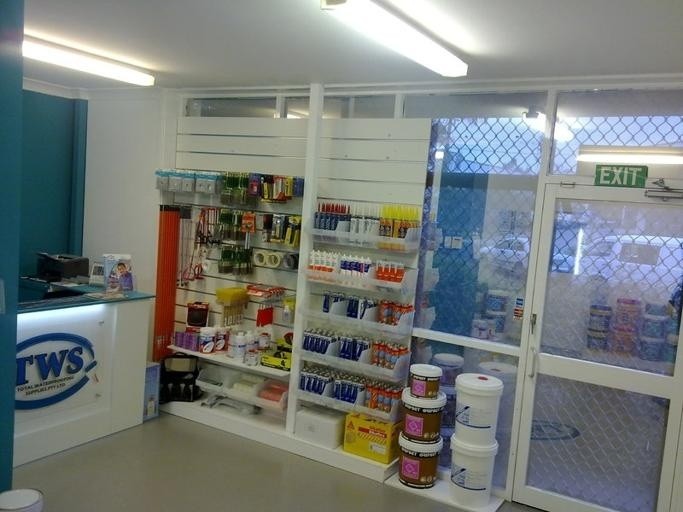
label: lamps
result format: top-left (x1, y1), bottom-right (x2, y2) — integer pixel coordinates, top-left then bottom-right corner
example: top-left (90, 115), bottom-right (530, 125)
top-left (576, 149), bottom-right (683, 165)
top-left (22, 34), bottom-right (154, 87)
top-left (319, 1), bottom-right (469, 77)
top-left (521, 108), bottom-right (575, 142)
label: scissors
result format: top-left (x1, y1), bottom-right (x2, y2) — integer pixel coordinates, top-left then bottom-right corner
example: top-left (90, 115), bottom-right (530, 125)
top-left (194, 252), bottom-right (204, 276)
top-left (183, 251), bottom-right (195, 280)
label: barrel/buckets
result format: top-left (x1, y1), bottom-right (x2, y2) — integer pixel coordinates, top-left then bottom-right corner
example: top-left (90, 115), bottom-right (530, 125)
top-left (586, 297), bottom-right (679, 365)
top-left (398, 288), bottom-right (508, 507)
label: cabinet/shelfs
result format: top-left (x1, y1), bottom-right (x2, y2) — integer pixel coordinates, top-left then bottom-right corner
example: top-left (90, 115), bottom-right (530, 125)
top-left (297, 227), bottom-right (421, 484)
top-left (159, 345), bottom-right (290, 452)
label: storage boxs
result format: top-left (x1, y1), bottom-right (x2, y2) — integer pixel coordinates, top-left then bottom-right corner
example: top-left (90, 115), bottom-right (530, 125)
top-left (344, 413), bottom-right (404, 464)
top-left (294, 405), bottom-right (344, 450)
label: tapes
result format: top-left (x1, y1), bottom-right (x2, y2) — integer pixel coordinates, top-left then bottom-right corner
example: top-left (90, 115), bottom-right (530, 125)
top-left (254, 250), bottom-right (299, 270)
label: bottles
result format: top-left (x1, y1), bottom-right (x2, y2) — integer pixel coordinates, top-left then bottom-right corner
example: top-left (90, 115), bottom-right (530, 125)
top-left (311, 199), bottom-right (424, 250)
top-left (299, 364), bottom-right (404, 414)
top-left (307, 248), bottom-right (408, 294)
top-left (224, 327), bottom-right (270, 364)
top-left (303, 324), bottom-right (412, 372)
top-left (320, 287), bottom-right (414, 331)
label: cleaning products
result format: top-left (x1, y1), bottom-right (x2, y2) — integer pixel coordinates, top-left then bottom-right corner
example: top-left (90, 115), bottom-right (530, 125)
top-left (301, 201), bottom-right (422, 412)
top-left (234, 326), bottom-right (265, 362)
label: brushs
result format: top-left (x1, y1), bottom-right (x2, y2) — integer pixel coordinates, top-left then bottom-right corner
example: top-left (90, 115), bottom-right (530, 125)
top-left (216, 287), bottom-right (247, 326)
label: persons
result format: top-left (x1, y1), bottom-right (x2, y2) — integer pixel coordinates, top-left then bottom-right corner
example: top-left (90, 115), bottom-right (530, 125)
top-left (416, 216), bottom-right (479, 372)
top-left (111, 262), bottom-right (131, 290)
